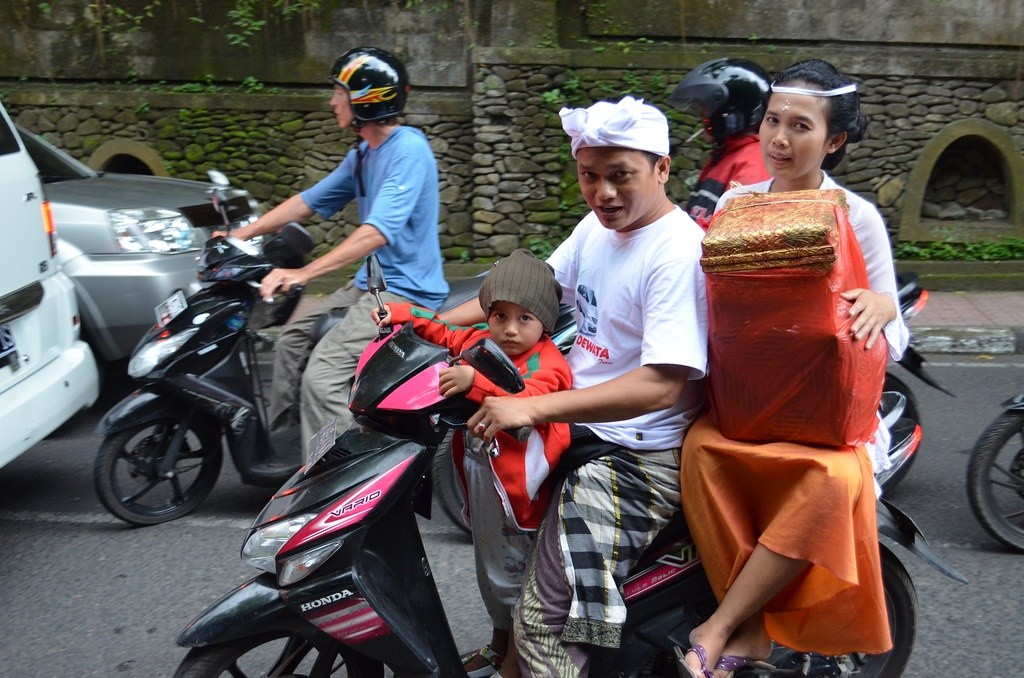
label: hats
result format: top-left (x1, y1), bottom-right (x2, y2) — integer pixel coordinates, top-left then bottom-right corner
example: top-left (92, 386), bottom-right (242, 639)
top-left (480, 247), bottom-right (563, 334)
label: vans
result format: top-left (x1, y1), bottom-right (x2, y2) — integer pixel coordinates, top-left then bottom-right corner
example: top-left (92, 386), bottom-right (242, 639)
top-left (0, 103), bottom-right (101, 474)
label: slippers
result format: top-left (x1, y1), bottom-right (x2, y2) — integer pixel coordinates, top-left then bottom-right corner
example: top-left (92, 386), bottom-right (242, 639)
top-left (673, 645), bottom-right (714, 678)
top-left (715, 655), bottom-right (753, 678)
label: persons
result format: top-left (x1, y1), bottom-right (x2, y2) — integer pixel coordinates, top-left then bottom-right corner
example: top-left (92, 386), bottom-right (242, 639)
top-left (674, 54), bottom-right (909, 678)
top-left (213, 45), bottom-right (450, 466)
top-left (668, 57), bottom-right (773, 234)
top-left (438, 95), bottom-right (711, 678)
top-left (370, 248), bottom-right (573, 678)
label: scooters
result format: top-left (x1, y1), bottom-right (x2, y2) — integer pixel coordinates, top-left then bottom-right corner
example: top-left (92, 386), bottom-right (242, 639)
top-left (171, 254), bottom-right (967, 678)
top-left (430, 269), bottom-right (958, 536)
top-left (93, 168), bottom-right (551, 529)
top-left (968, 391), bottom-right (1024, 556)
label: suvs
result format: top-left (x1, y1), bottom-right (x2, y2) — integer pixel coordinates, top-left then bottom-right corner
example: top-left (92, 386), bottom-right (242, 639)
top-left (11, 122), bottom-right (257, 436)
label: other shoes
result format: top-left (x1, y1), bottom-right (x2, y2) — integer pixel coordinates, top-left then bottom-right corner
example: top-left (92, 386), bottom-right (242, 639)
top-left (462, 645), bottom-right (505, 678)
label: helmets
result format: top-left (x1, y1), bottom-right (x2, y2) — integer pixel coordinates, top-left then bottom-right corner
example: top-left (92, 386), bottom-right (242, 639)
top-left (329, 46), bottom-right (409, 120)
top-left (668, 57), bottom-right (773, 138)
